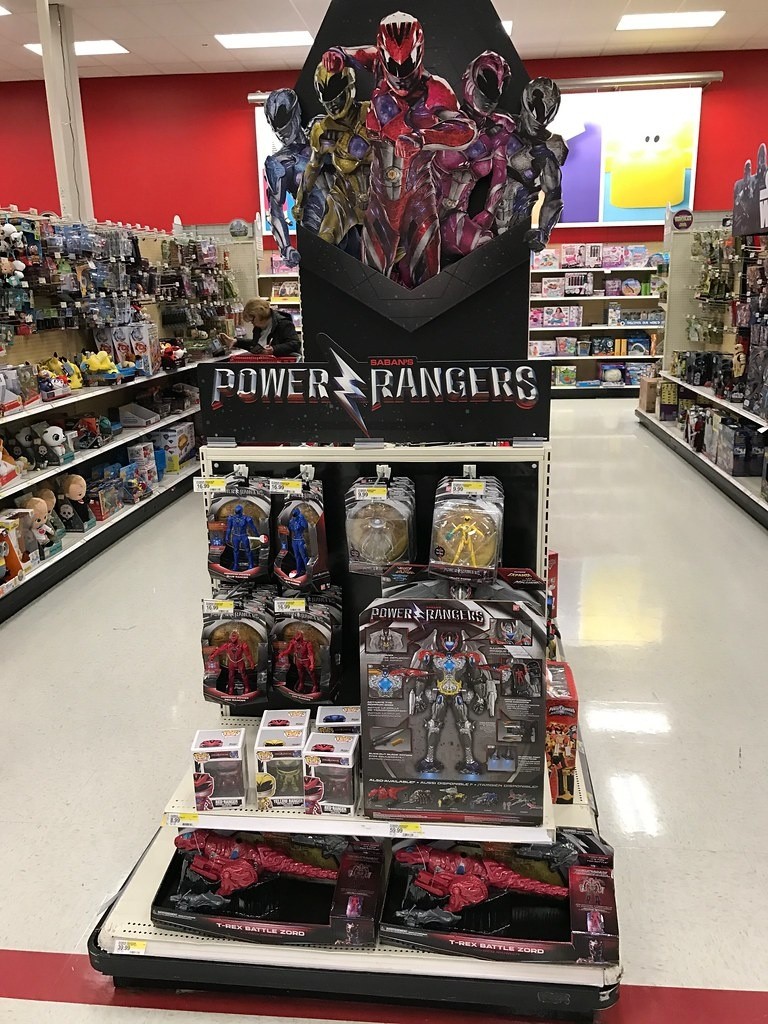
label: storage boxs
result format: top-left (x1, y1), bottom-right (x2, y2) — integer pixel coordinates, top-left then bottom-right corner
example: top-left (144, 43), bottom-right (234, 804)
top-left (87, 423), bottom-right (197, 521)
top-left (93, 319), bottom-right (161, 376)
top-left (530, 243), bottom-right (670, 389)
top-left (639, 377), bottom-right (768, 502)
top-left (270, 255), bottom-right (302, 331)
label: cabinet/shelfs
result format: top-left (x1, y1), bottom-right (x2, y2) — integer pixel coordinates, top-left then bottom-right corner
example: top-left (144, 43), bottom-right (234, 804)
top-left (529, 267), bottom-right (668, 399)
top-left (87, 438), bottom-right (626, 1023)
top-left (260, 273), bottom-right (302, 354)
top-left (0, 204), bottom-right (259, 619)
top-left (635, 202), bottom-right (768, 528)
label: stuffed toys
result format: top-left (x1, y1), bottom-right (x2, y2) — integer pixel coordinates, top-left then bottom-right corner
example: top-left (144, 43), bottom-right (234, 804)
top-left (43, 427), bottom-right (66, 463)
top-left (159, 341), bottom-right (187, 370)
top-left (37, 350), bottom-right (136, 388)
top-left (0, 223), bottom-right (25, 281)
top-left (16, 426), bottom-right (35, 460)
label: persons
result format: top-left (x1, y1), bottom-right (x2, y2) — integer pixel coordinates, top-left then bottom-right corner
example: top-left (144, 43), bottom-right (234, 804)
top-left (279, 630), bottom-right (319, 693)
top-left (36, 489), bottom-right (57, 530)
top-left (0, 261), bottom-right (15, 289)
top-left (551, 307), bottom-right (565, 324)
top-left (580, 273), bottom-right (594, 294)
top-left (732, 344), bottom-right (746, 391)
top-left (219, 298), bottom-right (302, 362)
top-left (577, 245), bottom-right (586, 263)
top-left (289, 509), bottom-right (313, 578)
top-left (733, 143), bottom-right (768, 235)
top-left (63, 474), bottom-right (90, 522)
top-left (208, 629), bottom-right (254, 695)
top-left (262, 11), bottom-right (569, 270)
top-left (449, 516), bottom-right (484, 569)
top-left (23, 498), bottom-right (55, 548)
top-left (225, 505), bottom-right (260, 570)
